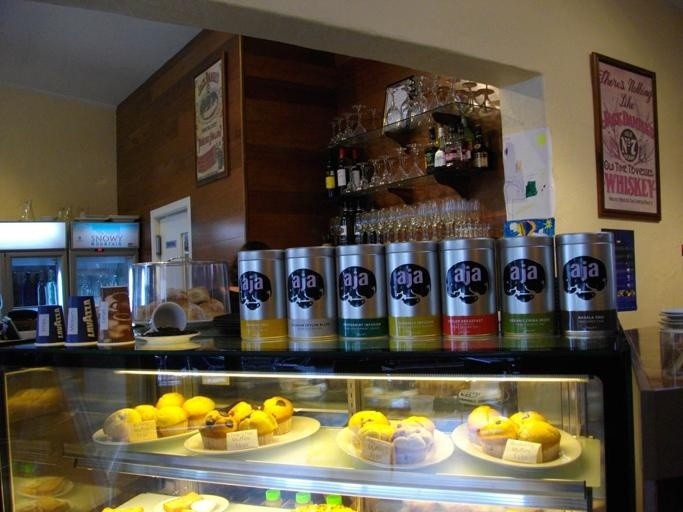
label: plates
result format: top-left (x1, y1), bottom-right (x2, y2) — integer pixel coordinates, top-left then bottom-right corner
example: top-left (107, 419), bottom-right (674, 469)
top-left (0, 338), bottom-right (36, 342)
top-left (92, 428), bottom-right (199, 445)
top-left (135, 331), bottom-right (202, 343)
top-left (154, 495), bottom-right (230, 512)
top-left (15, 499), bottom-right (75, 512)
top-left (336, 420), bottom-right (455, 469)
top-left (16, 480), bottom-right (74, 498)
top-left (452, 416), bottom-right (583, 473)
top-left (184, 415), bottom-right (321, 455)
top-left (658, 308), bottom-right (683, 328)
top-left (10, 409), bottom-right (77, 435)
top-left (137, 320), bottom-right (215, 329)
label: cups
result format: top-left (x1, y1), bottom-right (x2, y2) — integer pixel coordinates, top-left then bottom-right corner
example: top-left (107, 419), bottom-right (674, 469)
top-left (400, 79), bottom-right (420, 128)
top-left (342, 113), bottom-right (354, 139)
top-left (363, 107), bottom-right (382, 130)
top-left (328, 121), bottom-right (339, 148)
top-left (354, 197), bottom-right (481, 244)
top-left (330, 216), bottom-right (341, 245)
top-left (335, 118), bottom-right (344, 142)
top-left (444, 78), bottom-right (465, 116)
top-left (426, 72), bottom-right (445, 128)
top-left (392, 146), bottom-right (409, 182)
top-left (63, 296), bottom-right (99, 346)
top-left (344, 165), bottom-right (357, 192)
top-left (369, 159), bottom-right (382, 187)
top-left (405, 143), bottom-right (425, 178)
top-left (352, 105), bottom-right (368, 138)
top-left (385, 88), bottom-right (401, 127)
top-left (144, 302), bottom-right (187, 335)
top-left (356, 162), bottom-right (369, 190)
top-left (412, 76), bottom-right (431, 127)
top-left (455, 223), bottom-right (490, 238)
top-left (378, 155), bottom-right (392, 185)
top-left (97, 286), bottom-right (135, 346)
top-left (478, 84), bottom-right (498, 118)
top-left (461, 82), bottom-right (480, 115)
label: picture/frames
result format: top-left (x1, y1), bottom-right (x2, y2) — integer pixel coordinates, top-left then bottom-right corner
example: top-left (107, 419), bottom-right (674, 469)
top-left (192, 58), bottom-right (229, 186)
top-left (590, 52), bottom-right (665, 222)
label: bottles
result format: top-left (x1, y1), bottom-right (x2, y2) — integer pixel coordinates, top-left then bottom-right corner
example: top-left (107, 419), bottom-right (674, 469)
top-left (434, 122), bottom-right (446, 167)
top-left (284, 246), bottom-right (337, 350)
top-left (338, 201), bottom-right (353, 245)
top-left (34, 272), bottom-right (45, 306)
top-left (472, 122), bottom-right (490, 170)
top-left (555, 231), bottom-right (618, 351)
top-left (12, 271), bottom-right (22, 307)
top-left (324, 151), bottom-right (337, 201)
top-left (424, 126), bottom-right (438, 174)
top-left (238, 250), bottom-right (288, 351)
top-left (45, 270), bottom-right (58, 306)
top-left (457, 124), bottom-right (471, 163)
top-left (22, 272), bottom-right (35, 306)
top-left (335, 243), bottom-right (388, 350)
top-left (337, 149), bottom-right (350, 196)
top-left (351, 148), bottom-right (361, 191)
top-left (498, 236), bottom-right (556, 352)
top-left (444, 123), bottom-right (461, 168)
top-left (437, 238), bottom-right (498, 352)
top-left (354, 200), bottom-right (363, 218)
top-left (383, 241), bottom-right (441, 353)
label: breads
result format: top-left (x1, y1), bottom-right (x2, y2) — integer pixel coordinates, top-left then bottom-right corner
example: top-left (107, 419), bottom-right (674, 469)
top-left (156, 404), bottom-right (189, 437)
top-left (478, 421), bottom-right (518, 458)
top-left (516, 422), bottom-right (561, 464)
top-left (237, 409), bottom-right (278, 446)
top-left (198, 409), bottom-right (237, 451)
top-left (18, 496), bottom-right (70, 512)
top-left (157, 392), bottom-right (186, 411)
top-left (228, 399), bottom-right (258, 425)
top-left (347, 410), bottom-right (389, 450)
top-left (135, 403), bottom-right (155, 420)
top-left (510, 411), bottom-right (547, 426)
top-left (25, 473), bottom-right (65, 496)
top-left (182, 395), bottom-right (216, 432)
top-left (357, 422), bottom-right (395, 443)
top-left (260, 395), bottom-right (294, 435)
top-left (102, 407), bottom-right (141, 445)
top-left (397, 416), bottom-right (434, 437)
top-left (468, 405), bottom-right (502, 443)
top-left (390, 424), bottom-right (434, 465)
top-left (135, 285), bottom-right (225, 323)
top-left (163, 490), bottom-right (202, 512)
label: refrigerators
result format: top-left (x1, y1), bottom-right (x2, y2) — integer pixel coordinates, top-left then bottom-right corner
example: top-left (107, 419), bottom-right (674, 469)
top-left (69, 222), bottom-right (140, 306)
top-left (0, 220), bottom-right (69, 339)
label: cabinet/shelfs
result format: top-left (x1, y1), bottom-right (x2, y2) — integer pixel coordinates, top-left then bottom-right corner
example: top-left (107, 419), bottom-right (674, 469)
top-left (310, 96), bottom-right (502, 251)
top-left (0, 307), bottom-right (636, 512)
